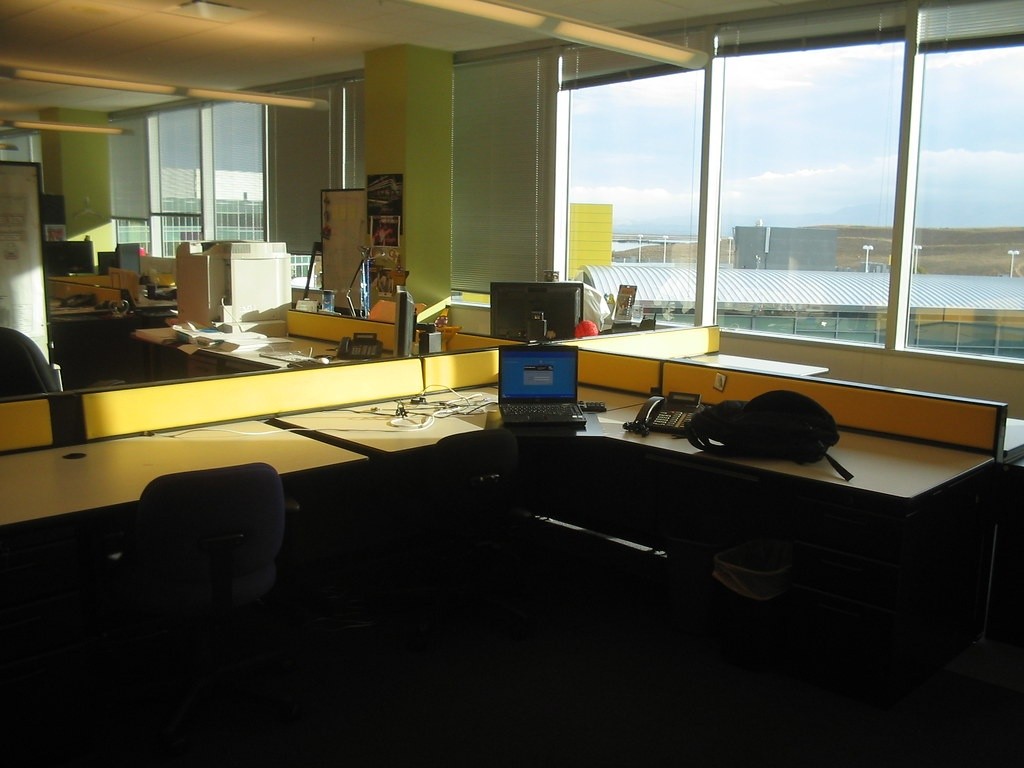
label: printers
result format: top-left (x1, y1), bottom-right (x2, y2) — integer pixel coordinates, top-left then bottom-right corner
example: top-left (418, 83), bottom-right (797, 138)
top-left (175, 239), bottom-right (292, 331)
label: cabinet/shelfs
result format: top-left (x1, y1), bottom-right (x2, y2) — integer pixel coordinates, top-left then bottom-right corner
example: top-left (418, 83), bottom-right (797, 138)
top-left (185, 353), bottom-right (220, 378)
top-left (0, 523), bottom-right (93, 768)
top-left (781, 495), bottom-right (991, 709)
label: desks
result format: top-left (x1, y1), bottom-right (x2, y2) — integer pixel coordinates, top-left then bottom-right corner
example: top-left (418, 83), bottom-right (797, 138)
top-left (135, 286), bottom-right (177, 311)
top-left (0, 420), bottom-right (370, 543)
top-left (270, 383), bottom-right (650, 465)
top-left (48, 296), bottom-right (119, 322)
top-left (688, 354), bottom-right (830, 376)
top-left (1003, 417), bottom-right (1024, 462)
top-left (134, 327), bottom-right (394, 366)
top-left (600, 402), bottom-right (998, 517)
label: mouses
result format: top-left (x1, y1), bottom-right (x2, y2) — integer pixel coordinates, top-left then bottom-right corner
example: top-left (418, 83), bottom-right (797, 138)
top-left (318, 358), bottom-right (329, 364)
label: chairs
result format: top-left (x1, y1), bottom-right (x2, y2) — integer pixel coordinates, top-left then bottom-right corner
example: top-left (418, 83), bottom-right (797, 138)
top-left (74, 461), bottom-right (304, 762)
top-left (369, 427), bottom-right (532, 653)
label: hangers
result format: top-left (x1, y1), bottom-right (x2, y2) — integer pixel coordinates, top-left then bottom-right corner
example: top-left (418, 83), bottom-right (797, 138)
top-left (75, 196), bottom-right (105, 219)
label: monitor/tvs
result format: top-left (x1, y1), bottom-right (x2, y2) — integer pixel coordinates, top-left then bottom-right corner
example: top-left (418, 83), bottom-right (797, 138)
top-left (115, 243), bottom-right (141, 275)
top-left (45, 241), bottom-right (93, 276)
top-left (394, 290), bottom-right (441, 357)
top-left (490, 281), bottom-right (584, 341)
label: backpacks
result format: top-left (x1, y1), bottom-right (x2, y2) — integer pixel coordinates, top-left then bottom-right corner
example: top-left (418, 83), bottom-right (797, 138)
top-left (685, 390), bottom-right (854, 481)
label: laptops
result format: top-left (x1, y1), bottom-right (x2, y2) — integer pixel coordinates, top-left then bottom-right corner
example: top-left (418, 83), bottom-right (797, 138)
top-left (498, 345), bottom-right (588, 427)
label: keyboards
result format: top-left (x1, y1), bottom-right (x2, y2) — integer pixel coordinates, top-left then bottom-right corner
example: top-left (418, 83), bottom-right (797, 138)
top-left (259, 352), bottom-right (316, 363)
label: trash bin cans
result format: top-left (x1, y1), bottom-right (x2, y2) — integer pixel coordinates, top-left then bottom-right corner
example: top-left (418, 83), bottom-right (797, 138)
top-left (710, 534), bottom-right (794, 671)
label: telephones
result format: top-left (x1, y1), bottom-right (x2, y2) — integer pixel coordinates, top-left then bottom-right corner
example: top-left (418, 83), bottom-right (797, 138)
top-left (597, 319), bottom-right (657, 335)
top-left (633, 389), bottom-right (711, 436)
top-left (52, 291), bottom-right (99, 309)
top-left (337, 332), bottom-right (383, 358)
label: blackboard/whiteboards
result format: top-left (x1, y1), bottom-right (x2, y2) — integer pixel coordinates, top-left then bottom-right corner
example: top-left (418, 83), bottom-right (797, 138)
top-left (320, 188), bottom-right (371, 317)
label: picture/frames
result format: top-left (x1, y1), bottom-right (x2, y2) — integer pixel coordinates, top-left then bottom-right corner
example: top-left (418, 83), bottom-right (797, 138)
top-left (45, 225), bottom-right (66, 241)
top-left (613, 284), bottom-right (638, 320)
